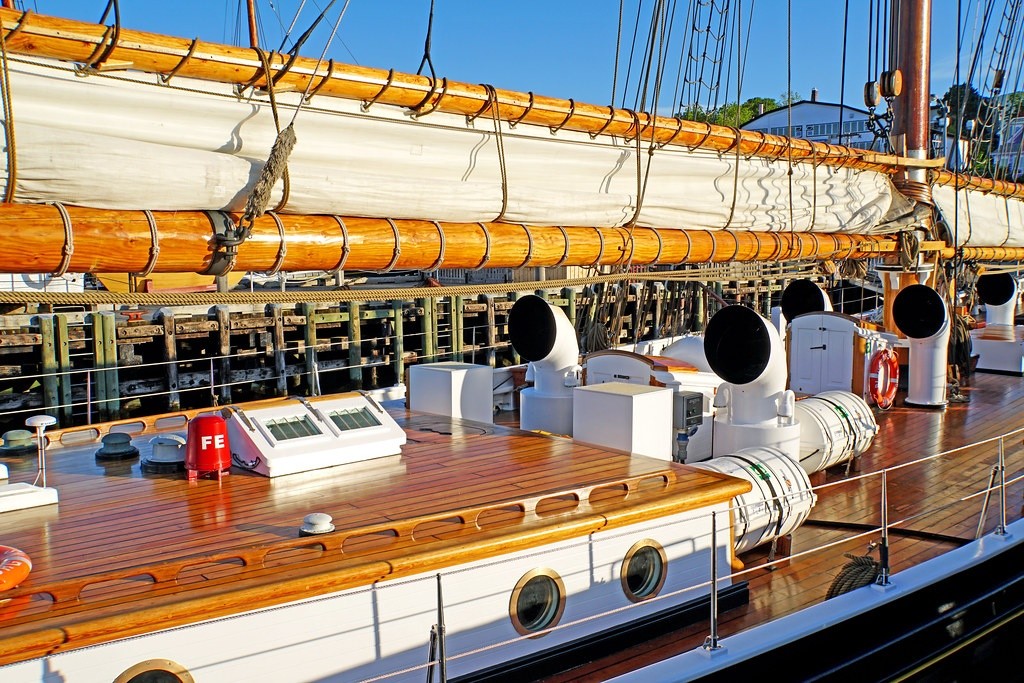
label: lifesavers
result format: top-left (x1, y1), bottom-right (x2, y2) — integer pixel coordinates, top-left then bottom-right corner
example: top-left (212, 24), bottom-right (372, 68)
top-left (867, 350), bottom-right (900, 407)
top-left (0, 543), bottom-right (32, 592)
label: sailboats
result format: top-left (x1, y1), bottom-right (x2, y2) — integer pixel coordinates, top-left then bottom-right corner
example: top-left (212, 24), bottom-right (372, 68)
top-left (0, 0), bottom-right (1024, 683)
